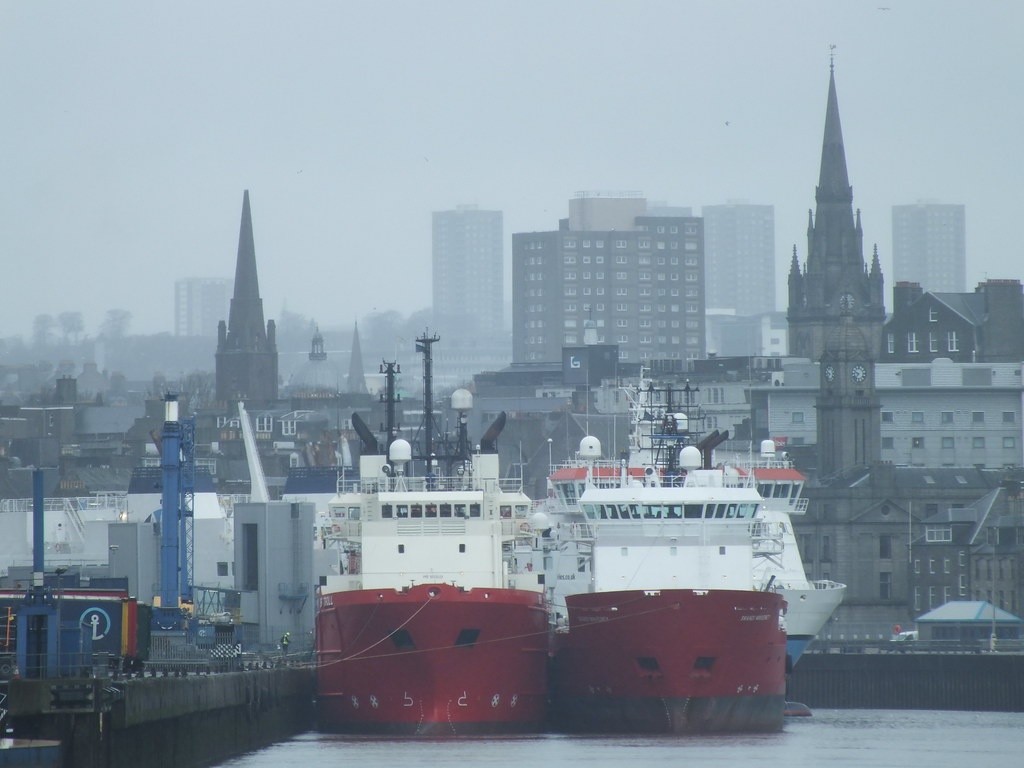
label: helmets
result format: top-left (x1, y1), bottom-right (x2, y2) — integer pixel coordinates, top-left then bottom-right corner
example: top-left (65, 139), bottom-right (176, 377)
top-left (286, 633), bottom-right (290, 636)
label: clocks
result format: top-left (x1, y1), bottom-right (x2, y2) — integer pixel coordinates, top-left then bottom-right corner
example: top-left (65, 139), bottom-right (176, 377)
top-left (838, 292), bottom-right (856, 310)
top-left (824, 365), bottom-right (836, 383)
top-left (852, 364), bottom-right (868, 383)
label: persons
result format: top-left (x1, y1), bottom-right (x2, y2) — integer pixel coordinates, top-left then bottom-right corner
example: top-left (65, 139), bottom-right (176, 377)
top-left (280, 632), bottom-right (291, 654)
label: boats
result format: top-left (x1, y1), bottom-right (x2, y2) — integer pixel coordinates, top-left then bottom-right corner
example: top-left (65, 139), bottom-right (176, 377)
top-left (312, 328), bottom-right (849, 740)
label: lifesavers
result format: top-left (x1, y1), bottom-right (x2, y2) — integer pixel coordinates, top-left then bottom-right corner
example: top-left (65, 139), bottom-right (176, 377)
top-left (520, 522), bottom-right (531, 533)
top-left (331, 524), bottom-right (340, 535)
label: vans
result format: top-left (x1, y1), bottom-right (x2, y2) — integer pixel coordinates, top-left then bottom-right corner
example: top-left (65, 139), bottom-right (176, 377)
top-left (891, 630), bottom-right (919, 642)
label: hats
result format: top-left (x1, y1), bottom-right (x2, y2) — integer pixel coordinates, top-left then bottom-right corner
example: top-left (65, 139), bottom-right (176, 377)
top-left (188, 600), bottom-right (192, 603)
